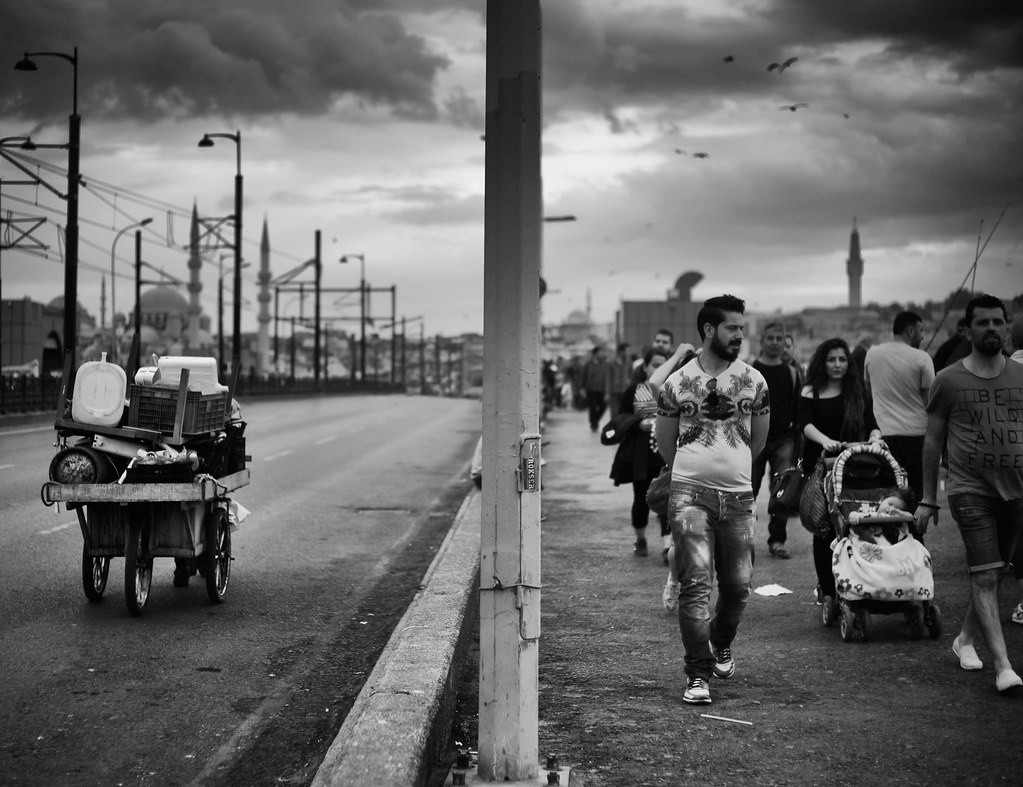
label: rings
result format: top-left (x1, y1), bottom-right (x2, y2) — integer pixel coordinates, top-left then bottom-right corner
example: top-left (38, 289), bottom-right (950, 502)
top-left (914, 516), bottom-right (918, 521)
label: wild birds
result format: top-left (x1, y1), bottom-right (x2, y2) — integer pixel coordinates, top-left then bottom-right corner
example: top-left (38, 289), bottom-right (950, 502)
top-left (766, 57), bottom-right (799, 73)
top-left (779, 103), bottom-right (808, 112)
top-left (694, 153), bottom-right (708, 158)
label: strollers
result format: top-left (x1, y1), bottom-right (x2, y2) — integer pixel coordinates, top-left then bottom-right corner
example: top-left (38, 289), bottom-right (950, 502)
top-left (812, 440), bottom-right (948, 640)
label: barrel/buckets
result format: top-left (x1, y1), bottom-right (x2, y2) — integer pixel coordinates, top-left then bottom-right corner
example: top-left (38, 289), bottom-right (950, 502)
top-left (72, 361), bottom-right (127, 427)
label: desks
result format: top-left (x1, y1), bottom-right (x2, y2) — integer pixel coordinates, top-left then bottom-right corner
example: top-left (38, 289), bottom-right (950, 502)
top-left (55, 347), bottom-right (243, 447)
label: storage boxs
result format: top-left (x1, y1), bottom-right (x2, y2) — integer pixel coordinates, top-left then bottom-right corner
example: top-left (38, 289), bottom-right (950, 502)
top-left (128, 383), bottom-right (246, 482)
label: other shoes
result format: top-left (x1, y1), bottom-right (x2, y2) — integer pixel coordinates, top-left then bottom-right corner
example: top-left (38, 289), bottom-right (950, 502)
top-left (172, 555), bottom-right (196, 585)
top-left (995, 669), bottom-right (1023, 691)
top-left (1012, 603), bottom-right (1023, 623)
top-left (771, 543), bottom-right (790, 556)
top-left (814, 588), bottom-right (822, 604)
top-left (952, 636), bottom-right (983, 669)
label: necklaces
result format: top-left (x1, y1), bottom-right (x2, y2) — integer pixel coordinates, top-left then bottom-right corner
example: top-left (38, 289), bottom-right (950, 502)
top-left (698, 355), bottom-right (731, 373)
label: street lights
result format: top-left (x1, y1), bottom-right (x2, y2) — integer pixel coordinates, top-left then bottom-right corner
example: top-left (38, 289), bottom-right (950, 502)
top-left (340, 253), bottom-right (368, 380)
top-left (14, 45), bottom-right (82, 406)
top-left (198, 126), bottom-right (245, 373)
top-left (110, 215), bottom-right (155, 364)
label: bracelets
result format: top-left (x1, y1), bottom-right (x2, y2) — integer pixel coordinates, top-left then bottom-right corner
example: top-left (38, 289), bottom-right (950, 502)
top-left (918, 502), bottom-right (941, 510)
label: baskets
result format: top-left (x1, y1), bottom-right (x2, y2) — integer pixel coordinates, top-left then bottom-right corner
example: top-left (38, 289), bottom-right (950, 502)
top-left (129, 382), bottom-right (228, 433)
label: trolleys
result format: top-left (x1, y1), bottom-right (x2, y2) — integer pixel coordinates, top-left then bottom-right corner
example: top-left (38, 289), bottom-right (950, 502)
top-left (78, 470), bottom-right (234, 615)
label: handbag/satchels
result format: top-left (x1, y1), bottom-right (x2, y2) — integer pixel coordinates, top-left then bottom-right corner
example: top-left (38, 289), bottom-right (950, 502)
top-left (600, 410), bottom-right (641, 447)
top-left (766, 465), bottom-right (804, 516)
top-left (799, 461), bottom-right (829, 535)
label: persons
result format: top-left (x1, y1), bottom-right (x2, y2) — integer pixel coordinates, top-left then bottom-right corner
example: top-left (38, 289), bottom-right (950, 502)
top-left (849, 490), bottom-right (914, 524)
top-left (751, 320), bottom-right (805, 560)
top-left (173, 396), bottom-right (244, 588)
top-left (542, 342), bottom-right (608, 433)
top-left (863, 311), bottom-right (936, 549)
top-left (851, 336), bottom-right (873, 363)
top-left (608, 329), bottom-right (703, 568)
top-left (656, 294), bottom-right (770, 704)
top-left (914, 294), bottom-right (1023, 690)
top-left (932, 317), bottom-right (973, 471)
top-left (1008, 316), bottom-right (1023, 364)
top-left (797, 337), bottom-right (889, 605)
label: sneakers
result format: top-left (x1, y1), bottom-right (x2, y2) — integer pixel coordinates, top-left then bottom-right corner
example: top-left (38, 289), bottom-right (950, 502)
top-left (709, 640), bottom-right (736, 679)
top-left (633, 536), bottom-right (649, 555)
top-left (683, 677), bottom-right (711, 704)
top-left (661, 547), bottom-right (672, 566)
top-left (662, 572), bottom-right (682, 612)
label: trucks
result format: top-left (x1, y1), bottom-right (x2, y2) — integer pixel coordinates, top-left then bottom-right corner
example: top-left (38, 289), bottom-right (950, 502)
top-left (1, 358), bottom-right (40, 378)
